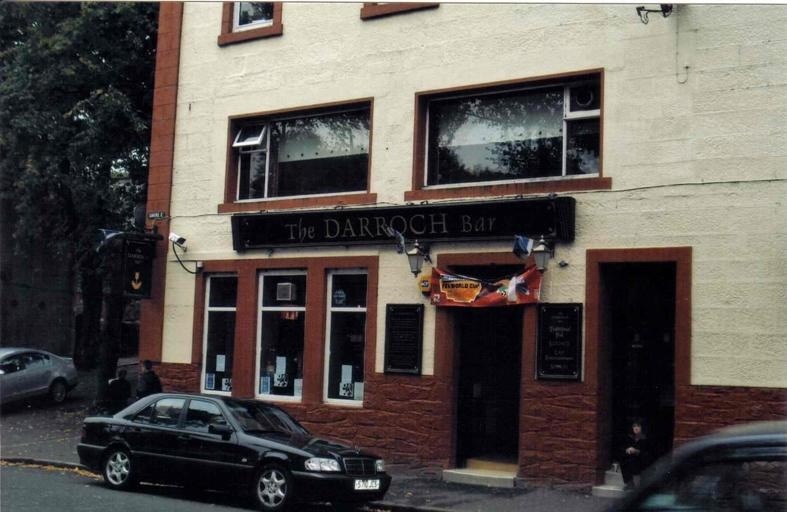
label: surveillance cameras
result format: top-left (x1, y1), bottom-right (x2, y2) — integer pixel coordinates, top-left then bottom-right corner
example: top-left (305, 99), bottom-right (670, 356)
top-left (168, 232), bottom-right (185, 245)
top-left (148, 212), bottom-right (166, 219)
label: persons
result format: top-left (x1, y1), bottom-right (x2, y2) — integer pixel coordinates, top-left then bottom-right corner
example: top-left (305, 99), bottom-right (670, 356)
top-left (617, 417), bottom-right (657, 491)
top-left (103, 369), bottom-right (130, 415)
top-left (135, 360), bottom-right (162, 399)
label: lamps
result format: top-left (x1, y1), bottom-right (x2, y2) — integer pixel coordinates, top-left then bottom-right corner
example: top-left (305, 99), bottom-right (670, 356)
top-left (531, 234), bottom-right (555, 274)
top-left (404, 238), bottom-right (432, 279)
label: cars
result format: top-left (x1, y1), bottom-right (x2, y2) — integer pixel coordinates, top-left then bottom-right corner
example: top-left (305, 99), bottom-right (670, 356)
top-left (1, 348), bottom-right (81, 406)
top-left (602, 421), bottom-right (786, 510)
top-left (78, 392), bottom-right (392, 510)
